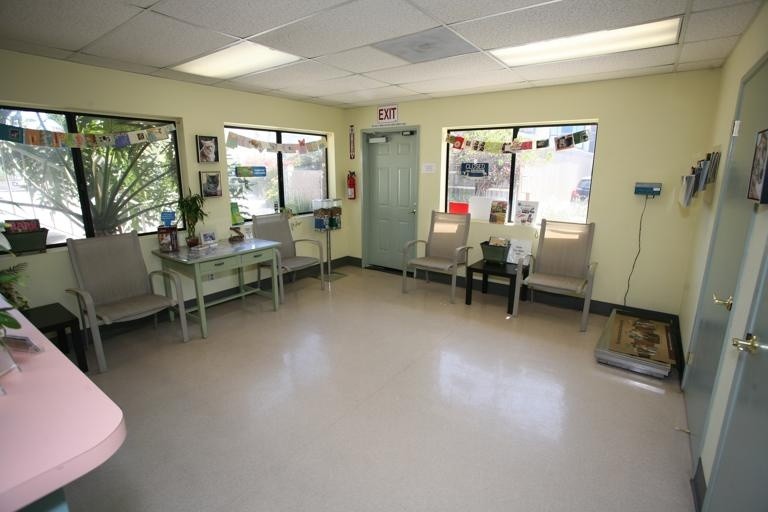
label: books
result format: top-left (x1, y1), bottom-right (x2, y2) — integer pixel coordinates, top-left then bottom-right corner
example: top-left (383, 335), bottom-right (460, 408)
top-left (4, 219), bottom-right (40, 232)
top-left (489, 201), bottom-right (507, 224)
top-left (192, 245), bottom-right (209, 250)
top-left (678, 151), bottom-right (720, 208)
top-left (506, 239), bottom-right (532, 265)
top-left (489, 236), bottom-right (509, 247)
top-left (515, 199), bottom-right (538, 225)
top-left (158, 225), bottom-right (177, 252)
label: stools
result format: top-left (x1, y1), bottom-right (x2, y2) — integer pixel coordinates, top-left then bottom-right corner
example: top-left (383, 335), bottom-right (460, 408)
top-left (24, 301), bottom-right (88, 372)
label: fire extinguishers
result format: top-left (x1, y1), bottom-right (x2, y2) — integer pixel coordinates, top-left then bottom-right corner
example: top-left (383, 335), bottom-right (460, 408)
top-left (347, 170), bottom-right (356, 199)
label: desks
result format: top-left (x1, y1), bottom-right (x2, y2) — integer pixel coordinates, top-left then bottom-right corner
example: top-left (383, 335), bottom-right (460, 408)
top-left (464, 260), bottom-right (529, 315)
top-left (151, 236), bottom-right (283, 339)
top-left (0, 293), bottom-right (129, 511)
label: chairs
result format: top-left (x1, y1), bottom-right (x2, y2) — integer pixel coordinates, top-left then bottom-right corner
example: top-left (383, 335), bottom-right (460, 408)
top-left (64, 231), bottom-right (189, 372)
top-left (510, 218), bottom-right (599, 332)
top-left (395, 209), bottom-right (474, 303)
top-left (250, 210), bottom-right (324, 302)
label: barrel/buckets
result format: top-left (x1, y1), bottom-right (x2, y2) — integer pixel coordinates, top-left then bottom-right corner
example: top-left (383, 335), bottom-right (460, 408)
top-left (312, 198), bottom-right (343, 218)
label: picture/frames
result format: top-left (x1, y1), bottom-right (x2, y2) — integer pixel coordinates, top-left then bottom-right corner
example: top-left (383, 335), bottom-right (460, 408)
top-left (195, 135), bottom-right (220, 164)
top-left (198, 171), bottom-right (224, 197)
top-left (200, 229), bottom-right (218, 245)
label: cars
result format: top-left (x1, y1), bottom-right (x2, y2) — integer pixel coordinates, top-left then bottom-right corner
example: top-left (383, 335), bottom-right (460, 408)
top-left (569, 178), bottom-right (591, 204)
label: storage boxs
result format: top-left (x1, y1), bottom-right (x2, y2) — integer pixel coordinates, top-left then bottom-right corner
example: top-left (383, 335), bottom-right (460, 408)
top-left (480, 240), bottom-right (509, 266)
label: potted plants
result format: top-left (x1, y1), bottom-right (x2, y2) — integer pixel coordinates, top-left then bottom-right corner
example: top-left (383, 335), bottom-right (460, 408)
top-left (175, 187), bottom-right (209, 245)
